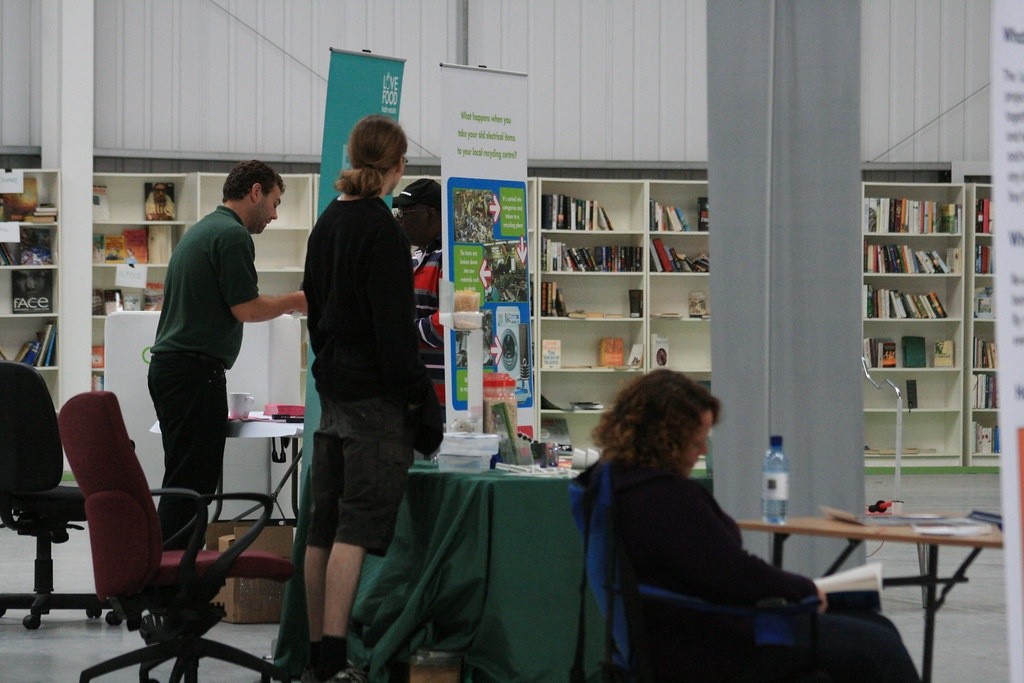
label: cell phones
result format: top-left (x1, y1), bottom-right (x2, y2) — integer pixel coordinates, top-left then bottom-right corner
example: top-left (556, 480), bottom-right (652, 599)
top-left (518, 323), bottom-right (530, 380)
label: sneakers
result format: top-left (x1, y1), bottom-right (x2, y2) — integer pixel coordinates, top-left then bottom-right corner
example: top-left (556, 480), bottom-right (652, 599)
top-left (300, 656), bottom-right (370, 683)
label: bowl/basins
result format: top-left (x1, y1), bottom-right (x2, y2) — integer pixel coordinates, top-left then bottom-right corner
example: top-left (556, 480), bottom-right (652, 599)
top-left (277, 404), bottom-right (306, 417)
top-left (454, 311), bottom-right (485, 329)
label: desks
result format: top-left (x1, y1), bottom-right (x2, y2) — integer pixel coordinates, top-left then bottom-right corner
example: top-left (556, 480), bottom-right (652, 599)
top-left (733, 516), bottom-right (1003, 683)
top-left (271, 470), bottom-right (714, 683)
top-left (149, 411), bottom-right (305, 527)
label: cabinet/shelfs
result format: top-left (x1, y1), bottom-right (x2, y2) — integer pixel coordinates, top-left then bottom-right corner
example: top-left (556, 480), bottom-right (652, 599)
top-left (0, 168), bottom-right (999, 476)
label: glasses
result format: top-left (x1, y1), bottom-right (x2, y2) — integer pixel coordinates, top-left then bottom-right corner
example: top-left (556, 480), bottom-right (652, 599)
top-left (393, 207), bottom-right (432, 220)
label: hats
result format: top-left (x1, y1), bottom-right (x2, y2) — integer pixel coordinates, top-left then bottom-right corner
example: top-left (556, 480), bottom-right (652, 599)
top-left (391, 178), bottom-right (442, 210)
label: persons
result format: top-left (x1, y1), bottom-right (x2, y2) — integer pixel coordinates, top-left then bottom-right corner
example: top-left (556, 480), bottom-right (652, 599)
top-left (301, 114), bottom-right (440, 683)
top-left (570, 370), bottom-right (921, 683)
top-left (392, 178), bottom-right (446, 423)
top-left (149, 159), bottom-right (307, 552)
top-left (146, 182), bottom-right (174, 220)
top-left (15, 270), bottom-right (46, 298)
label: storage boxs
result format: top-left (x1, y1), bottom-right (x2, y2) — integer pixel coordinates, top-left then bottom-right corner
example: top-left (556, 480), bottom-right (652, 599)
top-left (438, 433), bottom-right (499, 474)
top-left (206, 522), bottom-right (294, 624)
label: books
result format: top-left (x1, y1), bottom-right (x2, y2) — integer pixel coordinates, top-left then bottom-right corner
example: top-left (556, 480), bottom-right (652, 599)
top-left (627, 344), bottom-right (642, 368)
top-left (971, 198), bottom-right (999, 454)
top-left (542, 193), bottom-right (642, 271)
top-left (811, 561), bottom-right (882, 614)
top-left (864, 445), bottom-right (918, 454)
top-left (600, 337), bottom-right (624, 366)
top-left (864, 285), bottom-right (947, 318)
top-left (863, 337), bottom-right (954, 367)
top-left (661, 287), bottom-right (711, 321)
top-left (542, 280), bottom-right (623, 318)
top-left (651, 333), bottom-right (668, 369)
top-left (865, 197), bottom-right (962, 271)
top-left (650, 197), bottom-right (710, 272)
top-left (542, 339), bottom-right (561, 368)
top-left (0, 178), bottom-right (175, 392)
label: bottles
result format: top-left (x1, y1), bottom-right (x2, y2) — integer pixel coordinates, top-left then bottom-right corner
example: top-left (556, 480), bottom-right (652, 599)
top-left (483, 373), bottom-right (518, 439)
top-left (892, 499), bottom-right (903, 516)
top-left (762, 436), bottom-right (789, 525)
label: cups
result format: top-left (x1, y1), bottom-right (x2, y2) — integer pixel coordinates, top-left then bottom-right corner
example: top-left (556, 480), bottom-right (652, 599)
top-left (570, 448), bottom-right (599, 470)
top-left (454, 292), bottom-right (480, 312)
top-left (227, 393), bottom-right (254, 419)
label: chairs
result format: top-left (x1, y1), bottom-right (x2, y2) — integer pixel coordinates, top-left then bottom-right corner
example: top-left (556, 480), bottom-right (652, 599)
top-left (57, 391), bottom-right (295, 683)
top-left (0, 361), bottom-right (102, 631)
top-left (568, 461), bottom-right (824, 683)
